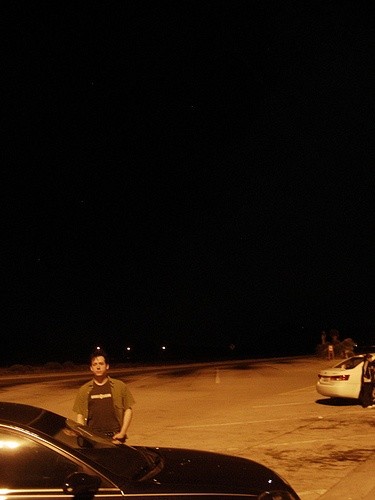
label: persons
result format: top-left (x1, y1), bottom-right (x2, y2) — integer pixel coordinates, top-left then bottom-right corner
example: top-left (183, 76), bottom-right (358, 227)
top-left (72, 351), bottom-right (136, 443)
top-left (319, 333), bottom-right (358, 359)
top-left (359, 354), bottom-right (375, 409)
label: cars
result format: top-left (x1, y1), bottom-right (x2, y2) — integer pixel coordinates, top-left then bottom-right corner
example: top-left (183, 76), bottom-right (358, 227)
top-left (316, 353), bottom-right (375, 404)
top-left (1, 401), bottom-right (300, 500)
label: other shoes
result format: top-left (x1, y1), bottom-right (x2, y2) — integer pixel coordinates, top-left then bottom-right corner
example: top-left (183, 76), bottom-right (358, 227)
top-left (367, 405), bottom-right (375, 409)
